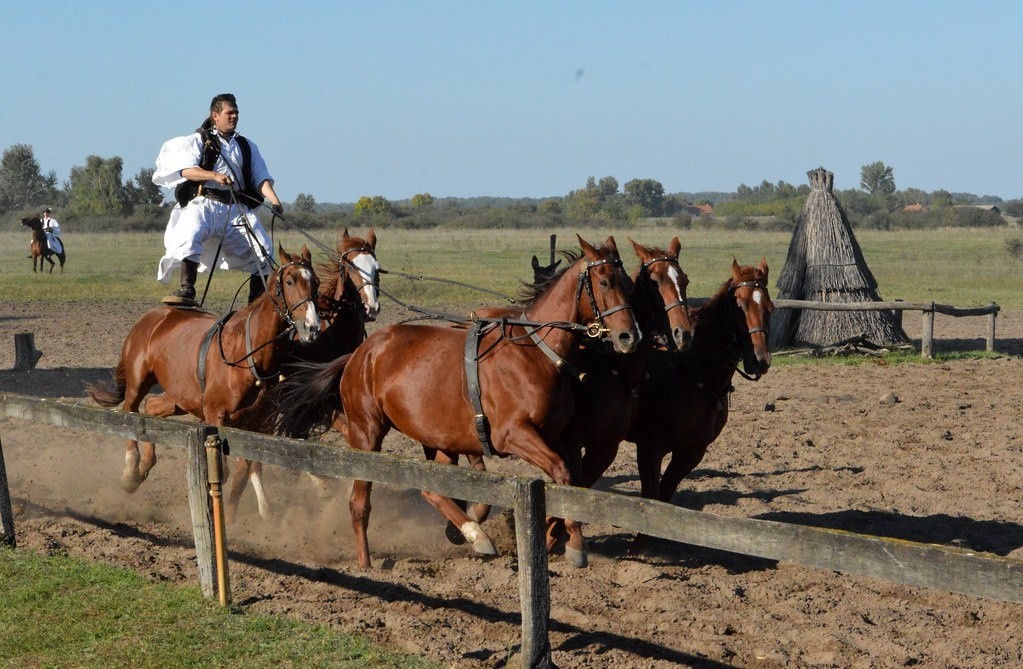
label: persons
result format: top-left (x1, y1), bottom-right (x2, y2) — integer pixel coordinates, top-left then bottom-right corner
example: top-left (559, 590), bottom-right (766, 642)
top-left (27, 208), bottom-right (62, 259)
top-left (151, 94), bottom-right (284, 307)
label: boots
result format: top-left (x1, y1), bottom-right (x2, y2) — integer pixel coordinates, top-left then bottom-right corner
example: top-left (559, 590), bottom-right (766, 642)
top-left (248, 274), bottom-right (269, 305)
top-left (163, 259), bottom-right (199, 305)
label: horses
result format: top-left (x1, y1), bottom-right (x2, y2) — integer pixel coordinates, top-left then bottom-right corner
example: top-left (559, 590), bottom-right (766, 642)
top-left (19, 213), bottom-right (66, 275)
top-left (248, 226), bottom-right (382, 521)
top-left (81, 240), bottom-right (322, 526)
top-left (463, 233), bottom-right (695, 567)
top-left (259, 232), bottom-right (642, 568)
top-left (635, 254), bottom-right (777, 502)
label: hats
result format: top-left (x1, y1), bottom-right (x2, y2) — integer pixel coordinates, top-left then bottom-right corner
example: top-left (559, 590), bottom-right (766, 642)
top-left (43, 208), bottom-right (50, 212)
